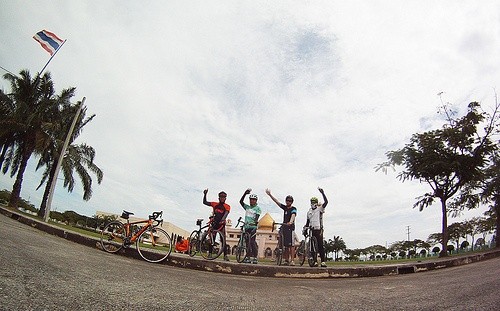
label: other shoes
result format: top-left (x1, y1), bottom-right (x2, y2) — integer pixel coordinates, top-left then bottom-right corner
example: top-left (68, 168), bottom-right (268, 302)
top-left (289, 260), bottom-right (295, 266)
top-left (223, 255), bottom-right (229, 262)
top-left (283, 261), bottom-right (289, 265)
top-left (244, 257), bottom-right (251, 263)
top-left (253, 259), bottom-right (258, 264)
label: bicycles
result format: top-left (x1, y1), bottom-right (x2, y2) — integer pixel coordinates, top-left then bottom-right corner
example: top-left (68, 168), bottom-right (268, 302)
top-left (297, 225), bottom-right (322, 266)
top-left (234, 216), bottom-right (259, 263)
top-left (100, 210), bottom-right (173, 262)
top-left (270, 220), bottom-right (295, 266)
top-left (186, 217), bottom-right (226, 260)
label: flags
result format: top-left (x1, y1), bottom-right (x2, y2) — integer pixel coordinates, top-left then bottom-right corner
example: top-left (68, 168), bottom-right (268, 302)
top-left (32, 29), bottom-right (65, 57)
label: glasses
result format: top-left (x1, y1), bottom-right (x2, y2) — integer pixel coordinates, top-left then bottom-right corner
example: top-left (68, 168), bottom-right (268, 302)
top-left (286, 200), bottom-right (292, 202)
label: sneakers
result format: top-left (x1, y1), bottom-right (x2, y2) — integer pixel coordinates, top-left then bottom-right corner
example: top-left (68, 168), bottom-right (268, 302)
top-left (314, 262), bottom-right (318, 267)
top-left (321, 262), bottom-right (327, 267)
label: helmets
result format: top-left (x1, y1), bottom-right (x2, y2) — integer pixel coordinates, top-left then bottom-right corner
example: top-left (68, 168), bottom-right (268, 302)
top-left (219, 192), bottom-right (227, 197)
top-left (286, 195), bottom-right (293, 200)
top-left (310, 197), bottom-right (318, 202)
top-left (249, 194), bottom-right (258, 200)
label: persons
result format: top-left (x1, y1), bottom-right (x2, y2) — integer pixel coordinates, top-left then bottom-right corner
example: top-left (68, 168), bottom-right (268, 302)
top-left (115, 225), bottom-right (160, 245)
top-left (175, 236), bottom-right (192, 255)
top-left (240, 188), bottom-right (261, 264)
top-left (302, 187), bottom-right (328, 267)
top-left (266, 188), bottom-right (297, 266)
top-left (203, 188), bottom-right (230, 261)
